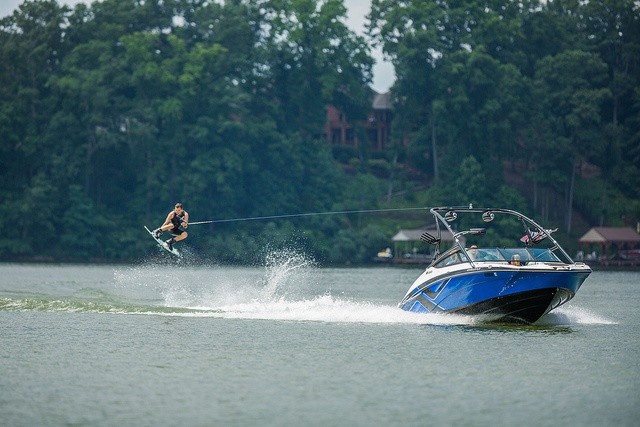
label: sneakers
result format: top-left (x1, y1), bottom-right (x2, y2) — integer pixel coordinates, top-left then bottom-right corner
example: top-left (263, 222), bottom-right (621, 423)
top-left (153, 228), bottom-right (162, 239)
top-left (166, 237), bottom-right (177, 250)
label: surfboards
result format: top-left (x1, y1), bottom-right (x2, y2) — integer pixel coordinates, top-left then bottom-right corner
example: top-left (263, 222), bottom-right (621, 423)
top-left (144, 226), bottom-right (179, 257)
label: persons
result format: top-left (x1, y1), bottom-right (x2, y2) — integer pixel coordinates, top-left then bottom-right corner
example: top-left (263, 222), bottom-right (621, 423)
top-left (153, 203), bottom-right (189, 250)
top-left (461, 245), bottom-right (479, 261)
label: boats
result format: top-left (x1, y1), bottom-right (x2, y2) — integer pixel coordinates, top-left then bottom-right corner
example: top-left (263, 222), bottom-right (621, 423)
top-left (371, 230), bottom-right (467, 267)
top-left (398, 203), bottom-right (592, 326)
